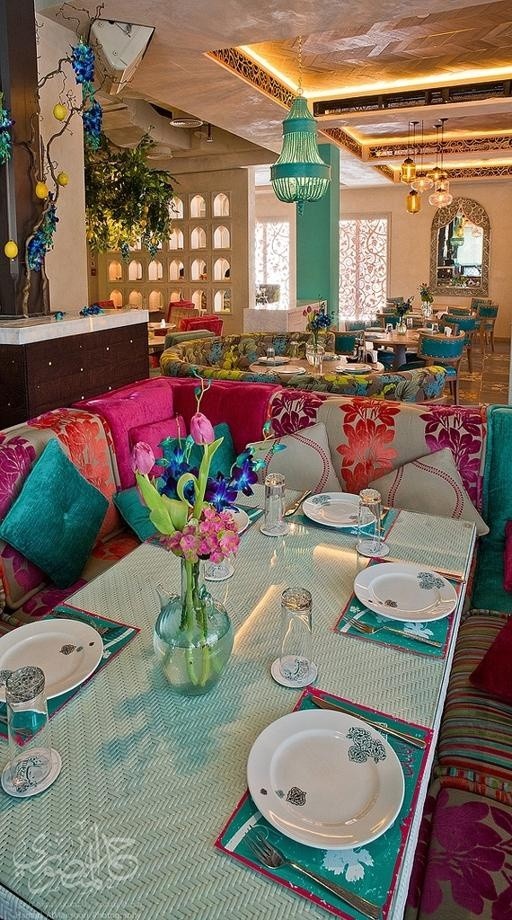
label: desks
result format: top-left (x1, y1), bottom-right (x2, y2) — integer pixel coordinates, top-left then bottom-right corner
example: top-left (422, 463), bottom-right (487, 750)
top-left (242, 298), bottom-right (327, 332)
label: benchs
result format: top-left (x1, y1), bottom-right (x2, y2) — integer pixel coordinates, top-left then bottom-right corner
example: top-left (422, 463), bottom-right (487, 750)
top-left (156, 330), bottom-right (448, 407)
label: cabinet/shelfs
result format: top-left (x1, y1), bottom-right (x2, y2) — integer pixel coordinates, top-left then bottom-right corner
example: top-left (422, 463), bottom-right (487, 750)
top-left (0, 321), bottom-right (148, 430)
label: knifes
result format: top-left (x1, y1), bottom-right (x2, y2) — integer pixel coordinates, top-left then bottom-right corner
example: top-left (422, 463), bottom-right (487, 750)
top-left (283, 488), bottom-right (311, 516)
top-left (311, 696), bottom-right (428, 751)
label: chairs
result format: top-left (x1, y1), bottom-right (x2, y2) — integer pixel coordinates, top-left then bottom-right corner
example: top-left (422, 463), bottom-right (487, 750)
top-left (88, 299), bottom-right (223, 378)
top-left (328, 297), bottom-right (499, 406)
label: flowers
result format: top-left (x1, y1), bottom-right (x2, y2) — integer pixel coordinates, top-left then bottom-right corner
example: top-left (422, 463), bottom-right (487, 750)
top-left (447, 269), bottom-right (469, 286)
top-left (415, 282), bottom-right (434, 303)
top-left (302, 294), bottom-right (335, 349)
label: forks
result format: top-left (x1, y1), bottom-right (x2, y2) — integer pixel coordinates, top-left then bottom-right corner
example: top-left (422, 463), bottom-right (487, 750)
top-left (50, 608), bottom-right (125, 635)
top-left (341, 610), bottom-right (444, 651)
top-left (243, 827), bottom-right (381, 919)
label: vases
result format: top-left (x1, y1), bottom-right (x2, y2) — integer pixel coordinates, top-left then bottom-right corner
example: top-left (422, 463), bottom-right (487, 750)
top-left (306, 336), bottom-right (325, 367)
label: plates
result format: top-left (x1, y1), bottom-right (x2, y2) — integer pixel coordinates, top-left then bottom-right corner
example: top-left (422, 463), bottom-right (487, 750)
top-left (269, 654), bottom-right (319, 687)
top-left (301, 491), bottom-right (376, 528)
top-left (353, 562), bottom-right (458, 622)
top-left (272, 365), bottom-right (305, 375)
top-left (343, 363), bottom-right (372, 373)
top-left (0, 746), bottom-right (62, 799)
top-left (204, 562), bottom-right (239, 582)
top-left (0, 618), bottom-right (103, 705)
top-left (245, 709), bottom-right (406, 850)
top-left (224, 504), bottom-right (249, 534)
top-left (355, 540), bottom-right (392, 558)
top-left (260, 523), bottom-right (288, 537)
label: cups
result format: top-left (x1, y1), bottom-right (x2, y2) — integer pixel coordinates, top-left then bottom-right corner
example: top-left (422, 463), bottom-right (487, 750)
top-left (279, 587), bottom-right (314, 680)
top-left (385, 318), bottom-right (450, 337)
top-left (5, 664), bottom-right (52, 785)
top-left (262, 472), bottom-right (288, 532)
top-left (356, 488), bottom-right (383, 554)
top-left (265, 347), bottom-right (275, 368)
top-left (206, 544), bottom-right (231, 577)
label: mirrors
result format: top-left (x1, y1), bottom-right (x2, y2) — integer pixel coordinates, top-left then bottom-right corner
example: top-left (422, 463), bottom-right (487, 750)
top-left (429, 196), bottom-right (490, 297)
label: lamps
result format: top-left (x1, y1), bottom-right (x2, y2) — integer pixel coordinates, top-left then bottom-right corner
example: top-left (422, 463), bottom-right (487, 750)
top-left (168, 112), bottom-right (204, 129)
top-left (402, 119), bottom-right (452, 214)
top-left (268, 35), bottom-right (331, 216)
top-left (454, 216), bottom-right (482, 238)
top-left (146, 145), bottom-right (173, 161)
